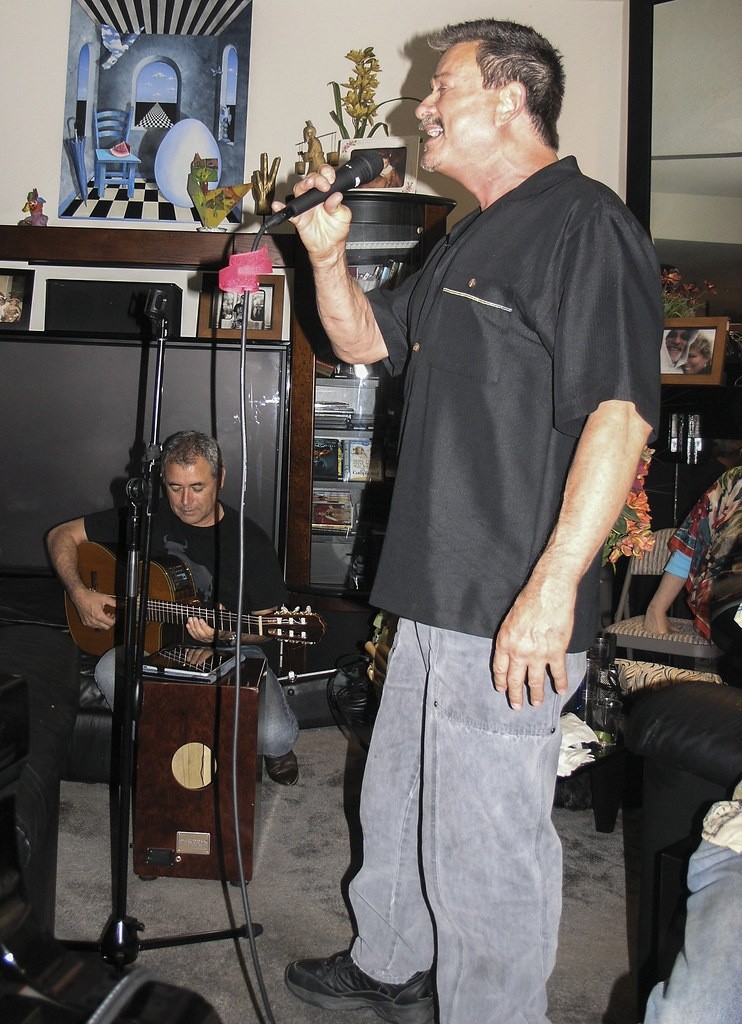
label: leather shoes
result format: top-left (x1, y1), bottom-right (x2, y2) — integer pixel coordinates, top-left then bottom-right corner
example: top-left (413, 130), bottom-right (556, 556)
top-left (264, 749), bottom-right (299, 786)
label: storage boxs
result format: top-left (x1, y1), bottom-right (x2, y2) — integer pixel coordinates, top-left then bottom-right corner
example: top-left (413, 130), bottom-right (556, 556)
top-left (46, 279), bottom-right (183, 337)
top-left (284, 190), bottom-right (456, 611)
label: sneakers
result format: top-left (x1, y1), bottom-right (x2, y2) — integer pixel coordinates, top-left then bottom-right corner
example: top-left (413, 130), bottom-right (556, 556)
top-left (285, 949), bottom-right (438, 1024)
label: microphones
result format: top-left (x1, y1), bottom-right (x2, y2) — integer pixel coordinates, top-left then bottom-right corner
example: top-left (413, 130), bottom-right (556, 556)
top-left (264, 149), bottom-right (385, 229)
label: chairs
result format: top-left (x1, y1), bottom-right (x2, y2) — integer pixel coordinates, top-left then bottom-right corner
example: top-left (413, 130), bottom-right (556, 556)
top-left (601, 528), bottom-right (725, 673)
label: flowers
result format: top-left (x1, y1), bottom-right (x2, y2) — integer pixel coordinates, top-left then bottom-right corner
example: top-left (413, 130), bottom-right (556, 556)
top-left (661, 268), bottom-right (719, 318)
top-left (327, 47), bottom-right (423, 143)
top-left (602, 445), bottom-right (655, 575)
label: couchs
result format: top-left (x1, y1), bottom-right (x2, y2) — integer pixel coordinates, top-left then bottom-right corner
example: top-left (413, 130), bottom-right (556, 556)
top-left (626, 679), bottom-right (742, 1023)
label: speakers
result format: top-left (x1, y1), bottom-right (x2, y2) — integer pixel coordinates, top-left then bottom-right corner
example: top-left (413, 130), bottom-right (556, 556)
top-left (134, 657), bottom-right (269, 887)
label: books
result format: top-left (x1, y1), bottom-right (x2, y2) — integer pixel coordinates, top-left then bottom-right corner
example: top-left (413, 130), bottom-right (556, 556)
top-left (310, 260), bottom-right (415, 590)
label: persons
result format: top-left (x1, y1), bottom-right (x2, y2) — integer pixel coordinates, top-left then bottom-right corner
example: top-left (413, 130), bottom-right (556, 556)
top-left (301, 120), bottom-right (326, 176)
top-left (622, 406), bottom-right (742, 1024)
top-left (359, 149), bottom-right (402, 189)
top-left (271, 18), bottom-right (660, 1024)
top-left (659, 328), bottom-right (712, 375)
top-left (46, 429), bottom-right (301, 785)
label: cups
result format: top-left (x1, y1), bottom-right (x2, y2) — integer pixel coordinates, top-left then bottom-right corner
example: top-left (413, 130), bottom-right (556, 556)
top-left (581, 638), bottom-right (622, 747)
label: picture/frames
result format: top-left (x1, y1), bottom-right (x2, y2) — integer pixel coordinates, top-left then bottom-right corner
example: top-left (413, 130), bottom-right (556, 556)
top-left (0, 268), bottom-right (35, 332)
top-left (339, 135), bottom-right (420, 194)
top-left (196, 273), bottom-right (285, 340)
top-left (660, 316), bottom-right (730, 387)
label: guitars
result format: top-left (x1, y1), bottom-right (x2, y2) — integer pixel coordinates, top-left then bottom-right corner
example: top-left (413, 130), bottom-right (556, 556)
top-left (59, 541), bottom-right (330, 657)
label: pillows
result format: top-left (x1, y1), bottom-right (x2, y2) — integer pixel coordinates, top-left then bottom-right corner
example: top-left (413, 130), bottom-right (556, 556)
top-left (613, 657), bottom-right (723, 697)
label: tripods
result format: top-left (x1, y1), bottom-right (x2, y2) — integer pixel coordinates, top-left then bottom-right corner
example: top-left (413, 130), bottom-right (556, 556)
top-left (52, 289), bottom-right (264, 982)
